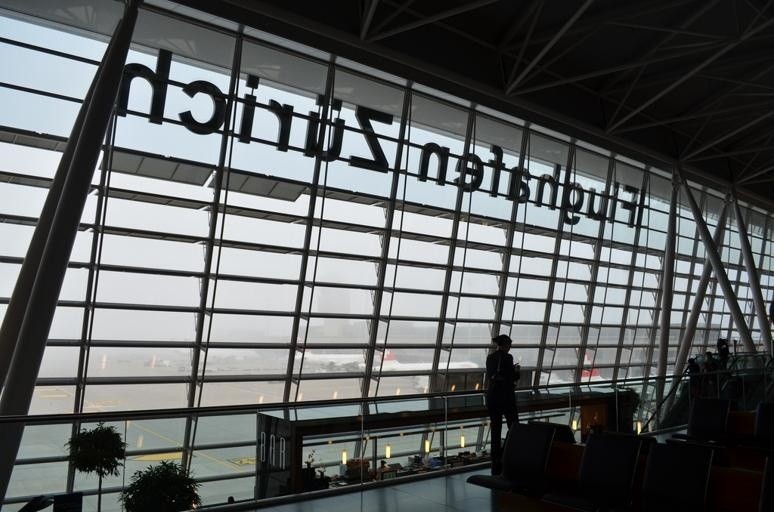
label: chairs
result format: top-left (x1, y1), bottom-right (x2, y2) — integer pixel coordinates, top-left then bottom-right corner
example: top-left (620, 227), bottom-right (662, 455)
top-left (465, 419), bottom-right (774, 512)
top-left (668, 395), bottom-right (773, 463)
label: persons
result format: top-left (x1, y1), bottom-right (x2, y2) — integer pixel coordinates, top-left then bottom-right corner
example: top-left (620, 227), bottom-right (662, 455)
top-left (701, 351), bottom-right (723, 398)
top-left (686, 357), bottom-right (701, 384)
top-left (690, 378), bottom-right (705, 401)
top-left (715, 338), bottom-right (731, 370)
top-left (483, 335), bottom-right (520, 477)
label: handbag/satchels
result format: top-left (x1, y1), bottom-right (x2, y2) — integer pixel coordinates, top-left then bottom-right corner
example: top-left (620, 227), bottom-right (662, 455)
top-left (485, 369), bottom-right (507, 392)
